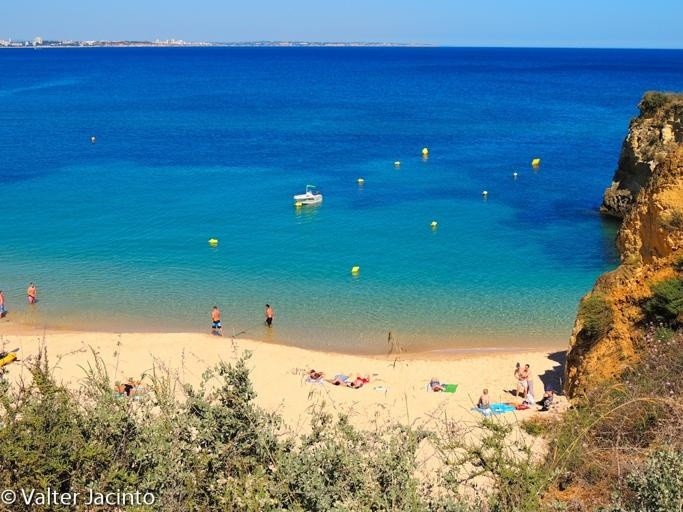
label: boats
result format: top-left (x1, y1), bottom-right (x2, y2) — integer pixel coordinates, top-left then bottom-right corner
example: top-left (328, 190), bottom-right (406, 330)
top-left (0, 350), bottom-right (18, 366)
top-left (293, 189), bottom-right (324, 206)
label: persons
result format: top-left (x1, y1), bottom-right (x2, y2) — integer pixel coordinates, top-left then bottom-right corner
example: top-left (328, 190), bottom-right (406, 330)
top-left (27, 281), bottom-right (37, 305)
top-left (512, 361), bottom-right (563, 412)
top-left (309, 369), bottom-right (324, 381)
top-left (345, 372), bottom-right (369, 388)
top-left (111, 376), bottom-right (153, 400)
top-left (262, 303), bottom-right (274, 328)
top-left (210, 305), bottom-right (224, 336)
top-left (428, 375), bottom-right (446, 392)
top-left (474, 388), bottom-right (491, 410)
top-left (0, 290), bottom-right (8, 320)
top-left (322, 370), bottom-right (353, 386)
